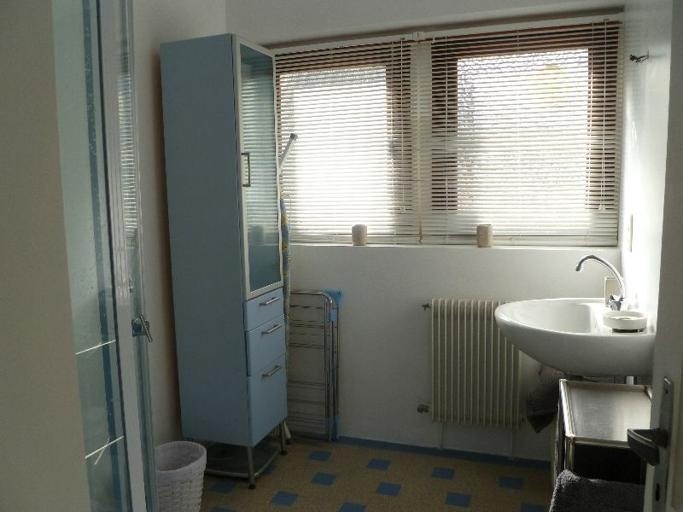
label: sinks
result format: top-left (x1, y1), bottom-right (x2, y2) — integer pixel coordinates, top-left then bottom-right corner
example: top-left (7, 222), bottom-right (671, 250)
top-left (494, 297), bottom-right (656, 377)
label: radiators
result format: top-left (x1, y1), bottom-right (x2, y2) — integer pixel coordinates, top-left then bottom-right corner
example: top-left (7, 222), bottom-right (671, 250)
top-left (417, 297), bottom-right (525, 429)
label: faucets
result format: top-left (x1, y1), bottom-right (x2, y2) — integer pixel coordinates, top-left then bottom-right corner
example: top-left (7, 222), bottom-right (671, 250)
top-left (575, 255), bottom-right (626, 295)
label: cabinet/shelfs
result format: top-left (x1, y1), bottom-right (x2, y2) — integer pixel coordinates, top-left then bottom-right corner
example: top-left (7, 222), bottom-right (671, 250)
top-left (158, 34), bottom-right (289, 301)
top-left (173, 288), bottom-right (291, 488)
top-left (551, 378), bottom-right (656, 486)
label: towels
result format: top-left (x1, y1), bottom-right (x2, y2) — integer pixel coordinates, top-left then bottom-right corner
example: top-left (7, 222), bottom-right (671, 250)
top-left (548, 469), bottom-right (645, 512)
top-left (521, 366), bottom-right (563, 433)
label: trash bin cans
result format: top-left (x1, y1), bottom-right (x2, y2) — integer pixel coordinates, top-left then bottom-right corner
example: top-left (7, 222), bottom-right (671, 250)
top-left (154, 440), bottom-right (207, 512)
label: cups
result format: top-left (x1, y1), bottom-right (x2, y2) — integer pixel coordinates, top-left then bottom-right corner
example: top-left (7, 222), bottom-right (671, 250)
top-left (604, 274), bottom-right (624, 307)
top-left (476, 223), bottom-right (493, 248)
top-left (352, 224), bottom-right (367, 246)
